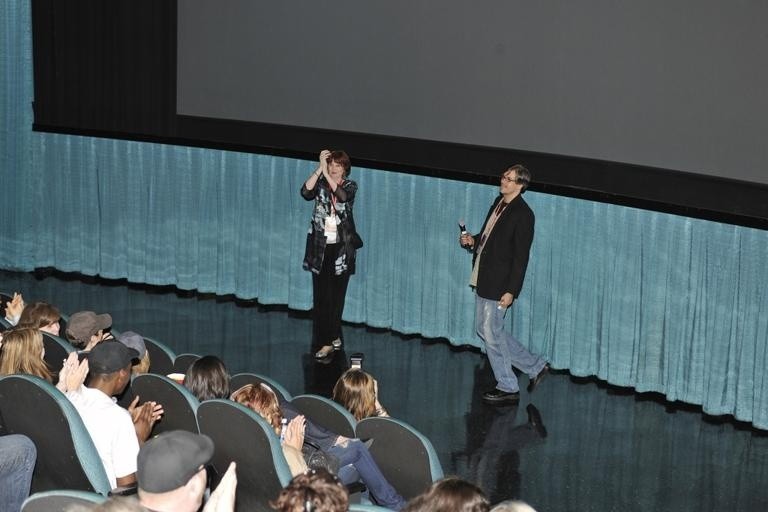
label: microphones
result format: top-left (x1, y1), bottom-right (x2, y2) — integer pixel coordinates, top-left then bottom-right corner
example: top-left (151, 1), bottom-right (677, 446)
top-left (458, 218), bottom-right (469, 248)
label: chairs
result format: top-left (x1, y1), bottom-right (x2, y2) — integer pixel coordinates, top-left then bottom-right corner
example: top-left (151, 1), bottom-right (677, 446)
top-left (76, 350), bottom-right (134, 399)
top-left (116, 372), bottom-right (199, 443)
top-left (136, 335), bottom-right (175, 378)
top-left (229, 371), bottom-right (293, 409)
top-left (196, 399), bottom-right (293, 512)
top-left (56, 312), bottom-right (68, 340)
top-left (356, 416), bottom-right (444, 485)
top-left (288, 393), bottom-right (357, 440)
top-left (174, 353), bottom-right (201, 372)
top-left (40, 329), bottom-right (79, 386)
top-left (21, 490), bottom-right (109, 511)
top-left (2, 371), bottom-right (113, 498)
top-left (1, 316), bottom-right (13, 334)
top-left (106, 326), bottom-right (120, 340)
top-left (2, 293), bottom-right (16, 317)
top-left (347, 502), bottom-right (395, 512)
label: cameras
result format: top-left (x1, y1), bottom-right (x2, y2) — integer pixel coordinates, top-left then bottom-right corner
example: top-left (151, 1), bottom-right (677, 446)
top-left (350, 353), bottom-right (364, 370)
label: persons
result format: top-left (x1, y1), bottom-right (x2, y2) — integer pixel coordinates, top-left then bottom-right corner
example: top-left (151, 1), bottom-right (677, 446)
top-left (459, 164), bottom-right (550, 406)
top-left (300, 148), bottom-right (359, 360)
top-left (0, 291), bottom-right (536, 512)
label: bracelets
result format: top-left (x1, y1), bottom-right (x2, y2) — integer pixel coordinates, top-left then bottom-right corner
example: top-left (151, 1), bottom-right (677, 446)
top-left (314, 171), bottom-right (321, 179)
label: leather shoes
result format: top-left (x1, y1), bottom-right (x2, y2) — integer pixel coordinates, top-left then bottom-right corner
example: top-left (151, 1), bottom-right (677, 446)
top-left (316, 339), bottom-right (342, 358)
top-left (527, 364), bottom-right (549, 393)
top-left (484, 388), bottom-right (520, 402)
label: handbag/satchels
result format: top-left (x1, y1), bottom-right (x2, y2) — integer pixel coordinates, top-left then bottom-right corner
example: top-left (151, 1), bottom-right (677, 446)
top-left (302, 440), bottom-right (328, 470)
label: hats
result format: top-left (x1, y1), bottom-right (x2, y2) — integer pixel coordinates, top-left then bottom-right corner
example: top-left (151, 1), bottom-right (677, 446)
top-left (66, 311), bottom-right (112, 344)
top-left (87, 330), bottom-right (146, 373)
top-left (137, 430), bottom-right (214, 494)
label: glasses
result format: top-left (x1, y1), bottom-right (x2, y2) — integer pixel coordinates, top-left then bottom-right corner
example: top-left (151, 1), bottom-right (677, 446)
top-left (498, 175), bottom-right (516, 182)
